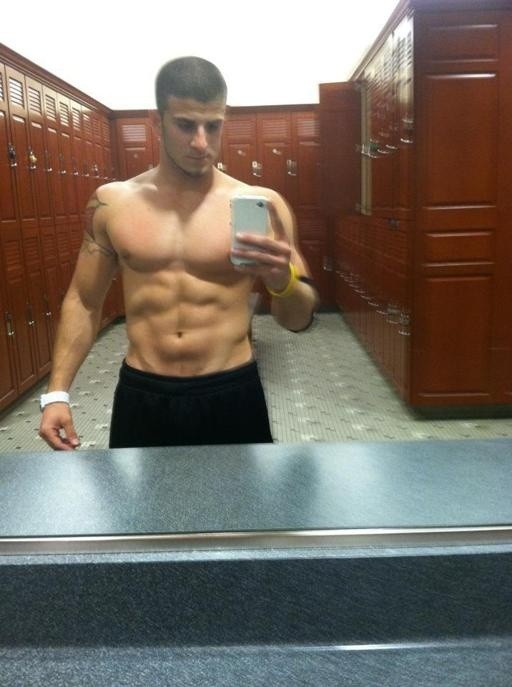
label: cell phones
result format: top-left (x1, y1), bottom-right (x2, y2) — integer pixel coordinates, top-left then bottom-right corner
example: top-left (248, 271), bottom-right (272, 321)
top-left (230, 194), bottom-right (269, 266)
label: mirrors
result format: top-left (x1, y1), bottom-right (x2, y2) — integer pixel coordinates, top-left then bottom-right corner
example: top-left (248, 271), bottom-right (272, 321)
top-left (0, 0), bottom-right (510, 553)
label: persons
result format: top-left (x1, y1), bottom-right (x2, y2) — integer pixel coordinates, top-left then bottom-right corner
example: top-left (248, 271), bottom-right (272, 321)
top-left (37, 56), bottom-right (317, 450)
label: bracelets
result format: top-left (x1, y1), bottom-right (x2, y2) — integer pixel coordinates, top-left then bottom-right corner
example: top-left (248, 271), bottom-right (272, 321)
top-left (265, 263), bottom-right (299, 298)
top-left (39, 391), bottom-right (71, 412)
